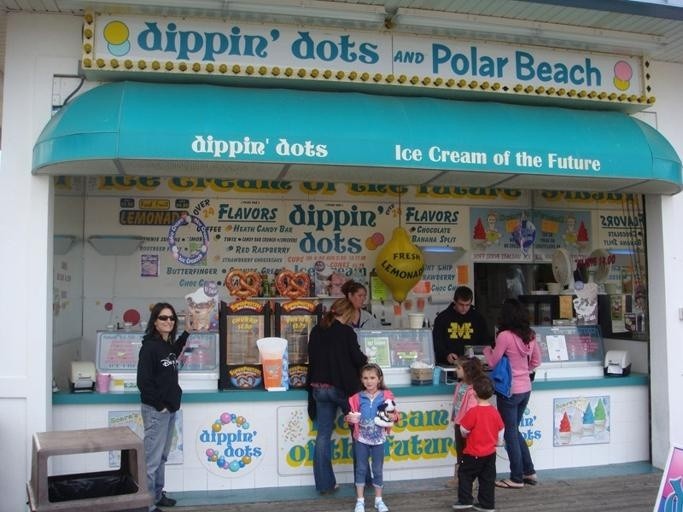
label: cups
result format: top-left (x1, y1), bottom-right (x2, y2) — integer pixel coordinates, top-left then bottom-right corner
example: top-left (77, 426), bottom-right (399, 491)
top-left (97, 371), bottom-right (110, 395)
top-left (256, 336), bottom-right (290, 391)
top-left (433, 366), bottom-right (442, 385)
top-left (349, 413), bottom-right (361, 424)
top-left (407, 312), bottom-right (425, 329)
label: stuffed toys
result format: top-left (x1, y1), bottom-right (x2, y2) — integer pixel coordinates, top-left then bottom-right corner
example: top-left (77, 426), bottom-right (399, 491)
top-left (372, 399), bottom-right (396, 428)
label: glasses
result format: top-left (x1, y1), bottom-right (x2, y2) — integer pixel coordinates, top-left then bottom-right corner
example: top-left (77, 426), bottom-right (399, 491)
top-left (157, 315), bottom-right (176, 320)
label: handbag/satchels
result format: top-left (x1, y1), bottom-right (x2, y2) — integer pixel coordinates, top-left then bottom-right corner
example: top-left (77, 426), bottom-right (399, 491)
top-left (529, 371), bottom-right (535, 381)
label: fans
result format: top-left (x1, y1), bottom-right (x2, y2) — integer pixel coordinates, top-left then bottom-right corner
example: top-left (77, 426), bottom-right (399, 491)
top-left (584, 249), bottom-right (612, 291)
top-left (549, 248), bottom-right (577, 295)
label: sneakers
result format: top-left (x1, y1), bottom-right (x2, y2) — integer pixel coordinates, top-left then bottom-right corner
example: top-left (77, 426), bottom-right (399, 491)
top-left (354, 498), bottom-right (364, 511)
top-left (152, 508), bottom-right (163, 511)
top-left (374, 498), bottom-right (388, 511)
top-left (451, 502), bottom-right (472, 508)
top-left (473, 503), bottom-right (495, 511)
top-left (157, 496), bottom-right (175, 505)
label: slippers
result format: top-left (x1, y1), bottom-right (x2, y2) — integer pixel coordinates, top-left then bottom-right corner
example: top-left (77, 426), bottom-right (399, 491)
top-left (494, 479), bottom-right (523, 488)
top-left (524, 478), bottom-right (536, 485)
top-left (322, 484), bottom-right (338, 496)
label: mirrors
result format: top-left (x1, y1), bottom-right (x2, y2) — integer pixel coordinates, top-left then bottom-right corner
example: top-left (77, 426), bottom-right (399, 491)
top-left (52, 173), bottom-right (82, 346)
top-left (530, 187), bottom-right (648, 342)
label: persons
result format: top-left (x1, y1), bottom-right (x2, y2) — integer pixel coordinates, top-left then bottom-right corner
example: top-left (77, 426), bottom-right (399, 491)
top-left (446, 357), bottom-right (482, 490)
top-left (135, 302), bottom-right (191, 512)
top-left (481, 297), bottom-right (541, 488)
top-left (451, 376), bottom-right (505, 512)
top-left (431, 286), bottom-right (488, 365)
top-left (340, 279), bottom-right (381, 329)
top-left (304, 298), bottom-right (373, 495)
top-left (341, 362), bottom-right (399, 511)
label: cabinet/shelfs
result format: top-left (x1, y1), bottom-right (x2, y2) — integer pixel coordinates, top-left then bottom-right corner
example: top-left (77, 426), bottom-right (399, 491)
top-left (493, 324), bottom-right (606, 381)
top-left (95, 329), bottom-right (221, 392)
top-left (353, 327), bottom-right (437, 388)
top-left (517, 294), bottom-right (573, 327)
top-left (596, 295), bottom-right (612, 335)
